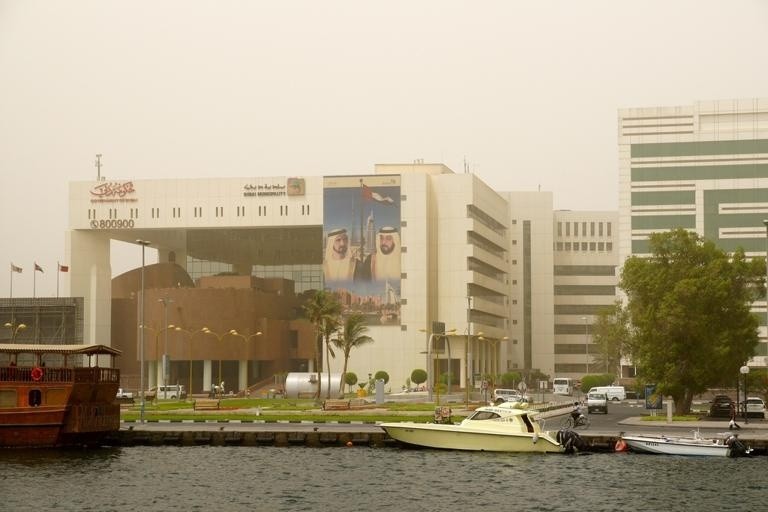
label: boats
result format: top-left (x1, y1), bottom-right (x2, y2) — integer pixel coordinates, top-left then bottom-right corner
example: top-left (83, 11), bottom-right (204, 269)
top-left (616, 429), bottom-right (734, 458)
top-left (0, 340), bottom-right (126, 450)
top-left (373, 401), bottom-right (568, 455)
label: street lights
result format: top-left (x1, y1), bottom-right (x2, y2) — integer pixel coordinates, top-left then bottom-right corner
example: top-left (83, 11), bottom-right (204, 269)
top-left (418, 293), bottom-right (512, 406)
top-left (739, 361), bottom-right (750, 424)
top-left (135, 238), bottom-right (152, 428)
top-left (3, 322), bottom-right (28, 342)
top-left (136, 297), bottom-right (265, 400)
top-left (581, 316), bottom-right (589, 374)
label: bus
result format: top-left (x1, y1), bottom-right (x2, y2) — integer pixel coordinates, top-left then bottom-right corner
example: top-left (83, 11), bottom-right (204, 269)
top-left (552, 377), bottom-right (574, 397)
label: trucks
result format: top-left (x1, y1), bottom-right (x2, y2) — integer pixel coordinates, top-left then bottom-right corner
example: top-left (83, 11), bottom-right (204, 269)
top-left (115, 387), bottom-right (134, 399)
top-left (585, 386), bottom-right (626, 402)
top-left (585, 392), bottom-right (609, 415)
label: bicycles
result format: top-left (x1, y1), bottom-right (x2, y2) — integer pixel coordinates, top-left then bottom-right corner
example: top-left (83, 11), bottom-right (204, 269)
top-left (558, 412), bottom-right (592, 431)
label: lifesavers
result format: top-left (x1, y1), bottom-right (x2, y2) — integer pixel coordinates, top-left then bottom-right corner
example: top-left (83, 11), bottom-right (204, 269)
top-left (31, 368), bottom-right (43, 380)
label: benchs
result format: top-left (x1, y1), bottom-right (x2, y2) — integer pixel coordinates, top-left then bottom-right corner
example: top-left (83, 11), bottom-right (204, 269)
top-left (192, 399), bottom-right (219, 409)
top-left (322, 399), bottom-right (351, 410)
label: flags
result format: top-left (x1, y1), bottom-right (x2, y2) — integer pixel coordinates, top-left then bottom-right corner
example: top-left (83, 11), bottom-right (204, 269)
top-left (57, 263), bottom-right (70, 273)
top-left (10, 264), bottom-right (24, 273)
top-left (34, 262), bottom-right (44, 274)
top-left (361, 183), bottom-right (394, 204)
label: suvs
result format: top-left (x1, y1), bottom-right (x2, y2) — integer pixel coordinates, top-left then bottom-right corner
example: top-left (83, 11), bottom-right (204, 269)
top-left (490, 388), bottom-right (528, 404)
top-left (707, 393), bottom-right (766, 418)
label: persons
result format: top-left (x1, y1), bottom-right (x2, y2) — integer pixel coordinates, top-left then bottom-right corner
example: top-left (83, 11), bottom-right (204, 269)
top-left (615, 436), bottom-right (627, 451)
top-left (322, 227), bottom-right (363, 283)
top-left (359, 225), bottom-right (400, 282)
top-left (570, 400), bottom-right (581, 422)
top-left (729, 403), bottom-right (742, 430)
